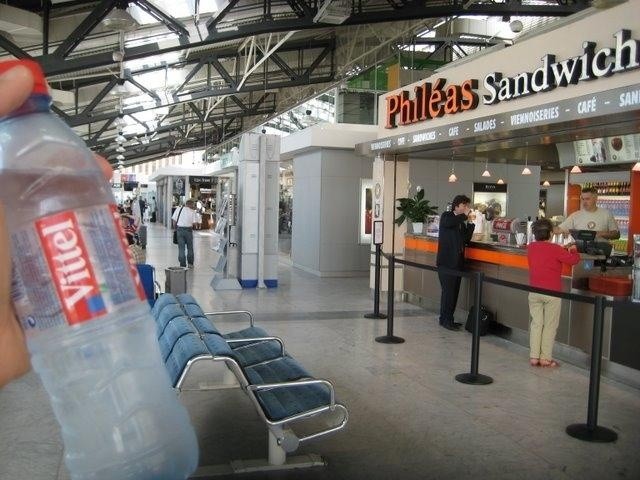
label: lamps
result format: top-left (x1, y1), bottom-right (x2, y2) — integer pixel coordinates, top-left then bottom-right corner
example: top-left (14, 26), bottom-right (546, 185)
top-left (112, 30), bottom-right (128, 173)
top-left (482, 154), bottom-right (491, 177)
top-left (447, 149), bottom-right (458, 182)
top-left (522, 148), bottom-right (531, 175)
top-left (570, 166), bottom-right (581, 174)
top-left (496, 160), bottom-right (504, 185)
top-left (542, 165), bottom-right (550, 186)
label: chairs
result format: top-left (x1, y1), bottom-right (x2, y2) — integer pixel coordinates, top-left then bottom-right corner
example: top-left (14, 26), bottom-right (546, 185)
top-left (148, 293), bottom-right (238, 479)
top-left (177, 293), bottom-right (348, 473)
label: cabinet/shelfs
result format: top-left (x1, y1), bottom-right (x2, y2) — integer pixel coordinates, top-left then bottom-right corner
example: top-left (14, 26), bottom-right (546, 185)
top-left (563, 162), bottom-right (640, 256)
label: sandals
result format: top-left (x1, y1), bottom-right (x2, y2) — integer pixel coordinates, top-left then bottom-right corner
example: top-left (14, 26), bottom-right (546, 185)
top-left (539, 359), bottom-right (561, 368)
top-left (529, 358), bottom-right (539, 367)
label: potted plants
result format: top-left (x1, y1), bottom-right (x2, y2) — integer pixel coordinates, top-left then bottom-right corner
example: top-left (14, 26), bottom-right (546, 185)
top-left (394, 188), bottom-right (439, 233)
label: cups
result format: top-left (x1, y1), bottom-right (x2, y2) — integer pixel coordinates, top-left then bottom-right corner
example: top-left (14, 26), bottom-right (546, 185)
top-left (515, 233), bottom-right (524, 244)
top-left (468, 212), bottom-right (476, 223)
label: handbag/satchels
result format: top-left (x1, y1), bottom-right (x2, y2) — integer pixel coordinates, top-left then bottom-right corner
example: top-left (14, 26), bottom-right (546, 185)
top-left (465, 305), bottom-right (494, 337)
top-left (173, 231), bottom-right (179, 244)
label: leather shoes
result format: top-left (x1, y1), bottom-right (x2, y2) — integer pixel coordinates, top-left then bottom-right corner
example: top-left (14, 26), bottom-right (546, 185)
top-left (439, 320), bottom-right (463, 331)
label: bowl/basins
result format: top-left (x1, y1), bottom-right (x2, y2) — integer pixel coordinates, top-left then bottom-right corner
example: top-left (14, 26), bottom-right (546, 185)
top-left (474, 233), bottom-right (484, 241)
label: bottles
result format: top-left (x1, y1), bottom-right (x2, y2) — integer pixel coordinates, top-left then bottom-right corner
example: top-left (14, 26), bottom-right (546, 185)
top-left (582, 181), bottom-right (630, 196)
top-left (611, 239), bottom-right (628, 251)
top-left (616, 220), bottom-right (629, 235)
top-left (596, 200), bottom-right (629, 217)
top-left (0, 60), bottom-right (200, 480)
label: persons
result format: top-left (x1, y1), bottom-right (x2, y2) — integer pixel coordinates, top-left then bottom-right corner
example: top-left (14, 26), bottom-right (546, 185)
top-left (171, 199), bottom-right (197, 269)
top-left (437, 195), bottom-right (475, 330)
top-left (195, 196), bottom-right (204, 229)
top-left (117, 195), bottom-right (156, 223)
top-left (0, 65), bottom-right (113, 390)
top-left (527, 219), bottom-right (579, 367)
top-left (552, 188), bottom-right (620, 243)
